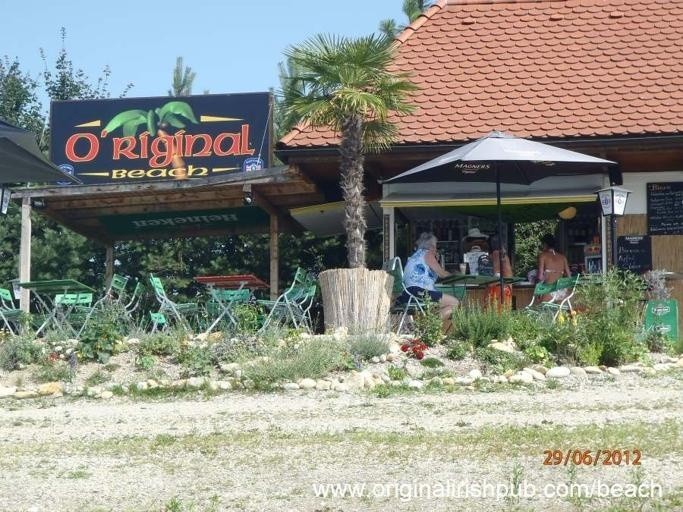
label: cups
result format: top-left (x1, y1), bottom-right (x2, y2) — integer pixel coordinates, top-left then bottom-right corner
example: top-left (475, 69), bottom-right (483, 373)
top-left (458, 263), bottom-right (467, 275)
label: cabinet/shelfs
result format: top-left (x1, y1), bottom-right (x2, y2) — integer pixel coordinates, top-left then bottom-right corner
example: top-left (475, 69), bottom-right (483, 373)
top-left (437, 240), bottom-right (459, 265)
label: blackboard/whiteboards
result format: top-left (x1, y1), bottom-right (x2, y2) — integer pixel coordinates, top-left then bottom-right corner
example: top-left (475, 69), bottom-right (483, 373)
top-left (617, 236), bottom-right (652, 275)
top-left (584, 255), bottom-right (602, 273)
top-left (646, 182), bottom-right (683, 235)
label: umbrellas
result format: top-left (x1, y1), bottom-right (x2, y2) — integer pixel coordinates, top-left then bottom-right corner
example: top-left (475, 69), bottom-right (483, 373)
top-left (382, 128), bottom-right (619, 305)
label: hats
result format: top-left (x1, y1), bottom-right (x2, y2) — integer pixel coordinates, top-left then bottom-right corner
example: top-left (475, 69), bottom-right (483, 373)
top-left (464, 227), bottom-right (489, 239)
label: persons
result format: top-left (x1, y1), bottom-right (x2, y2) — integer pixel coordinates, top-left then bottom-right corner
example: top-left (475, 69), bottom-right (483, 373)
top-left (399, 232), bottom-right (459, 320)
top-left (535, 233), bottom-right (572, 303)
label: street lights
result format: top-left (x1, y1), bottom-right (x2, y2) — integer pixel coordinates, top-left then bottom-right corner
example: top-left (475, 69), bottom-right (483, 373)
top-left (595, 182), bottom-right (633, 287)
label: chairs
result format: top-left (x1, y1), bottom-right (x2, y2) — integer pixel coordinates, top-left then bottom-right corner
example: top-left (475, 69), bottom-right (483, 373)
top-left (382, 256), bottom-right (439, 335)
top-left (525, 282), bottom-right (557, 310)
top-left (434, 286), bottom-right (465, 301)
top-left (536, 273), bottom-right (581, 326)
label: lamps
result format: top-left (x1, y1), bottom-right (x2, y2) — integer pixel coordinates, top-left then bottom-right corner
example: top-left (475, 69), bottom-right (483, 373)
top-left (31, 197), bottom-right (48, 209)
top-left (242, 184), bottom-right (256, 206)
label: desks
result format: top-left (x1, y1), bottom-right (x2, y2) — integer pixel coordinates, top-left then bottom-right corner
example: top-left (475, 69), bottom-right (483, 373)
top-left (435, 274), bottom-right (527, 335)
